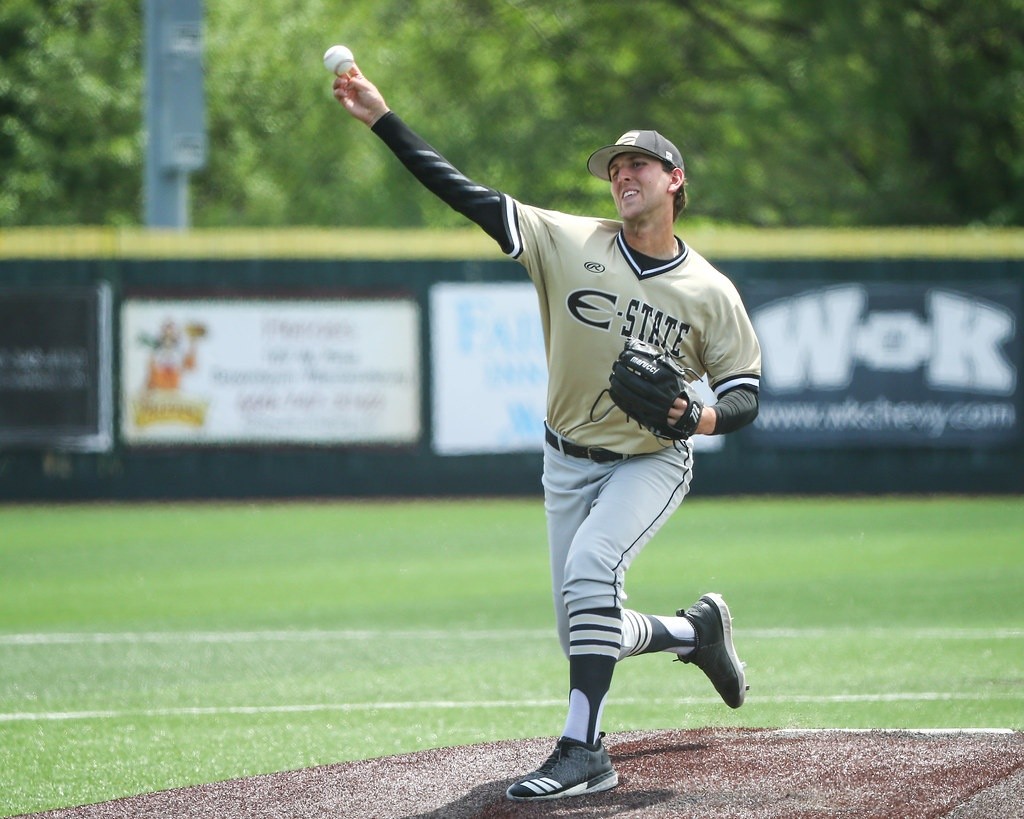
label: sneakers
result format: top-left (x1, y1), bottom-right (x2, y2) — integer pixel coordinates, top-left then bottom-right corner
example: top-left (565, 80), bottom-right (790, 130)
top-left (678, 593), bottom-right (745, 709)
top-left (506, 732), bottom-right (618, 801)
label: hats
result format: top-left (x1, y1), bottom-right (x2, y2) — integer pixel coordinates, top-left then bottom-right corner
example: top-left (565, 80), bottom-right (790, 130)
top-left (587, 130), bottom-right (684, 182)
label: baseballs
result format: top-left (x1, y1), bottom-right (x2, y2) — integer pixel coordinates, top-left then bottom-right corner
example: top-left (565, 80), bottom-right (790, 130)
top-left (323, 45), bottom-right (355, 77)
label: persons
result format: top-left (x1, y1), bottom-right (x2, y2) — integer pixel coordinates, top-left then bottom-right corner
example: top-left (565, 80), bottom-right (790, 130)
top-left (332, 63), bottom-right (762, 802)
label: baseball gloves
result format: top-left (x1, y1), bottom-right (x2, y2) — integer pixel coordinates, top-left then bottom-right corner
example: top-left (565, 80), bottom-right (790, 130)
top-left (606, 337), bottom-right (703, 443)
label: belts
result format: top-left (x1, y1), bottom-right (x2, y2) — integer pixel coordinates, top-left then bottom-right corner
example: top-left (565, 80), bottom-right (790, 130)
top-left (544, 422), bottom-right (643, 461)
